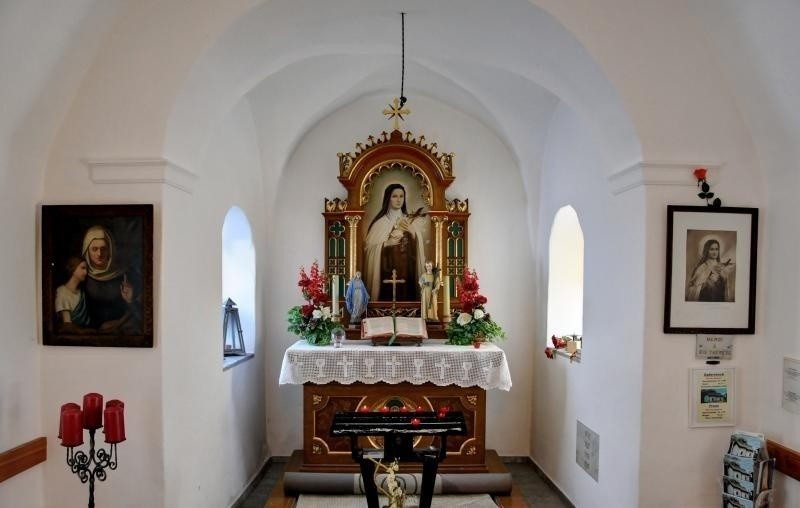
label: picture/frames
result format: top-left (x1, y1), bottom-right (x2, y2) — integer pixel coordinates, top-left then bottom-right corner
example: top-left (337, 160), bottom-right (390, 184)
top-left (662, 204), bottom-right (762, 335)
top-left (37, 202), bottom-right (156, 348)
top-left (688, 364), bottom-right (737, 428)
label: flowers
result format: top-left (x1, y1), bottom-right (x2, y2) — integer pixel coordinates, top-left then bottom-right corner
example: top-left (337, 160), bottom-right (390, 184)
top-left (693, 166), bottom-right (723, 206)
top-left (287, 263), bottom-right (338, 333)
top-left (450, 268), bottom-right (504, 344)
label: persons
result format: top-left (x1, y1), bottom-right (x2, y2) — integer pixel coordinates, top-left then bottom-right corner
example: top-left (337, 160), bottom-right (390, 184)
top-left (345, 271), bottom-right (373, 323)
top-left (417, 258), bottom-right (441, 321)
top-left (78, 224), bottom-right (137, 329)
top-left (53, 256), bottom-right (92, 327)
top-left (362, 183), bottom-right (429, 301)
top-left (684, 236), bottom-right (734, 301)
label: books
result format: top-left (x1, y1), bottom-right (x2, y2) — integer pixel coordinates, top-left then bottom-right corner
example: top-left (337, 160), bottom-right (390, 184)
top-left (721, 430), bottom-right (770, 507)
top-left (360, 316), bottom-right (429, 340)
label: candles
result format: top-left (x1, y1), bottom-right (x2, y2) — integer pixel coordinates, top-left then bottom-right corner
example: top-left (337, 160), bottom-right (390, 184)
top-left (359, 403), bottom-right (449, 426)
top-left (55, 391), bottom-right (127, 447)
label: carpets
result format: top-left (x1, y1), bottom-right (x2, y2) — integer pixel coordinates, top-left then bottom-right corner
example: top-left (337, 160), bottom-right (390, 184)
top-left (280, 471), bottom-right (512, 508)
